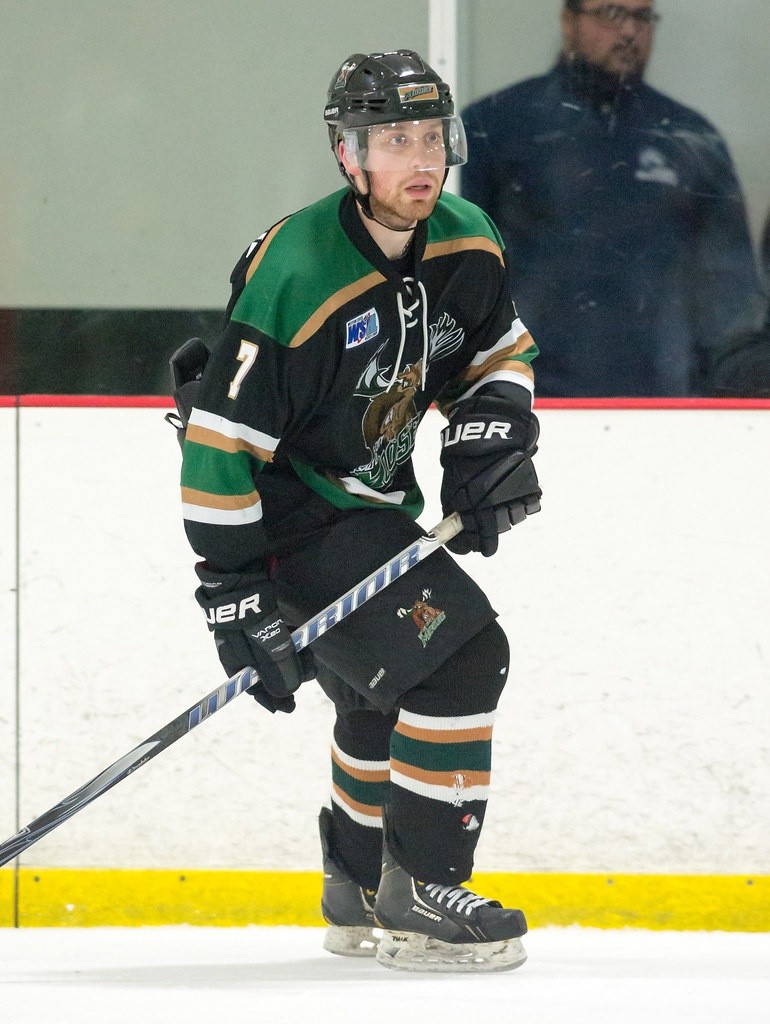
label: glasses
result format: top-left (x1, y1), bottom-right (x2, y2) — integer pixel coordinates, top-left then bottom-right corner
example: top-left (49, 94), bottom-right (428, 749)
top-left (573, 5), bottom-right (663, 26)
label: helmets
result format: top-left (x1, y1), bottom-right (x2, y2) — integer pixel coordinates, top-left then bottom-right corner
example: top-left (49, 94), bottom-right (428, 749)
top-left (324, 49), bottom-right (458, 170)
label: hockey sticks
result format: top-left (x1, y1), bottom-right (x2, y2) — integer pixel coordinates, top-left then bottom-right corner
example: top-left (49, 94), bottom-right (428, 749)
top-left (1, 511), bottom-right (464, 866)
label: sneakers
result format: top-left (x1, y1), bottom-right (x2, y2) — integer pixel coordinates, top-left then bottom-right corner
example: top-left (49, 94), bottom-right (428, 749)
top-left (319, 807), bottom-right (528, 973)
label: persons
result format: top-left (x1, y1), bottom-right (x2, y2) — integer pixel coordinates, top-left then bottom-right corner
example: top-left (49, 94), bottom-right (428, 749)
top-left (179, 49), bottom-right (541, 973)
top-left (460, 0), bottom-right (770, 398)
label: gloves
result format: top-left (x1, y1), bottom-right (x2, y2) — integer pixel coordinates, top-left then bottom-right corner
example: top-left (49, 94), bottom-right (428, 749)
top-left (439, 396), bottom-right (542, 557)
top-left (195, 561), bottom-right (320, 714)
